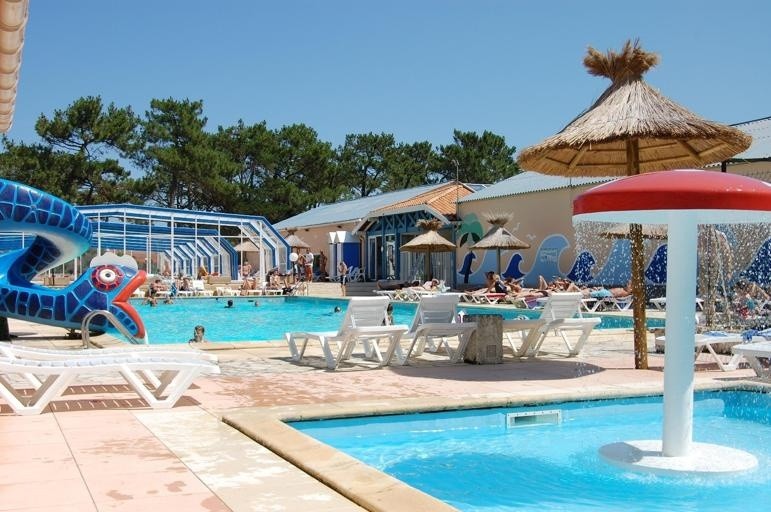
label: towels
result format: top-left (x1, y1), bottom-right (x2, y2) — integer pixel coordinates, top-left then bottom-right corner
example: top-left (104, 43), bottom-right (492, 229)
top-left (741, 330), bottom-right (771, 341)
top-left (702, 332), bottom-right (727, 336)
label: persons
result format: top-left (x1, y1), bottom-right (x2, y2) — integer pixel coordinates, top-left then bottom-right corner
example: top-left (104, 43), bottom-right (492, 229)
top-left (188, 324), bottom-right (211, 343)
top-left (255, 301), bottom-right (259, 306)
top-left (735, 275), bottom-right (771, 315)
top-left (143, 260), bottom-right (220, 306)
top-left (319, 250), bottom-right (328, 278)
top-left (240, 249), bottom-right (314, 294)
top-left (395, 271), bottom-right (633, 309)
top-left (381, 303), bottom-right (395, 325)
top-left (338, 261), bottom-right (347, 297)
top-left (224, 299), bottom-right (235, 309)
top-left (731, 274), bottom-right (755, 320)
top-left (699, 223), bottom-right (735, 329)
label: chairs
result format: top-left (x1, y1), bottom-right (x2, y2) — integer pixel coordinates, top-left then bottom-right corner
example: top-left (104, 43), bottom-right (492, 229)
top-left (0, 359), bottom-right (221, 415)
top-left (372, 281), bottom-right (633, 312)
top-left (656, 333), bottom-right (771, 372)
top-left (732, 342), bottom-right (771, 377)
top-left (518, 292), bottom-right (601, 357)
top-left (503, 319), bottom-right (547, 357)
top-left (128, 280), bottom-right (284, 297)
top-left (325, 266), bottom-right (365, 282)
top-left (361, 293), bottom-right (475, 366)
top-left (284, 296), bottom-right (408, 369)
top-left (650, 298), bottom-right (720, 311)
top-left (0, 342), bottom-right (218, 404)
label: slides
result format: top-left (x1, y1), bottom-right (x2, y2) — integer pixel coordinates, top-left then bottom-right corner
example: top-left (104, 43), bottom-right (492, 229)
top-left (0, 179), bottom-right (146, 340)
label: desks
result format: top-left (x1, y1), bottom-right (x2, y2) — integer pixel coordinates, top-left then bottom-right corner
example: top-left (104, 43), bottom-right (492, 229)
top-left (463, 314), bottom-right (503, 364)
